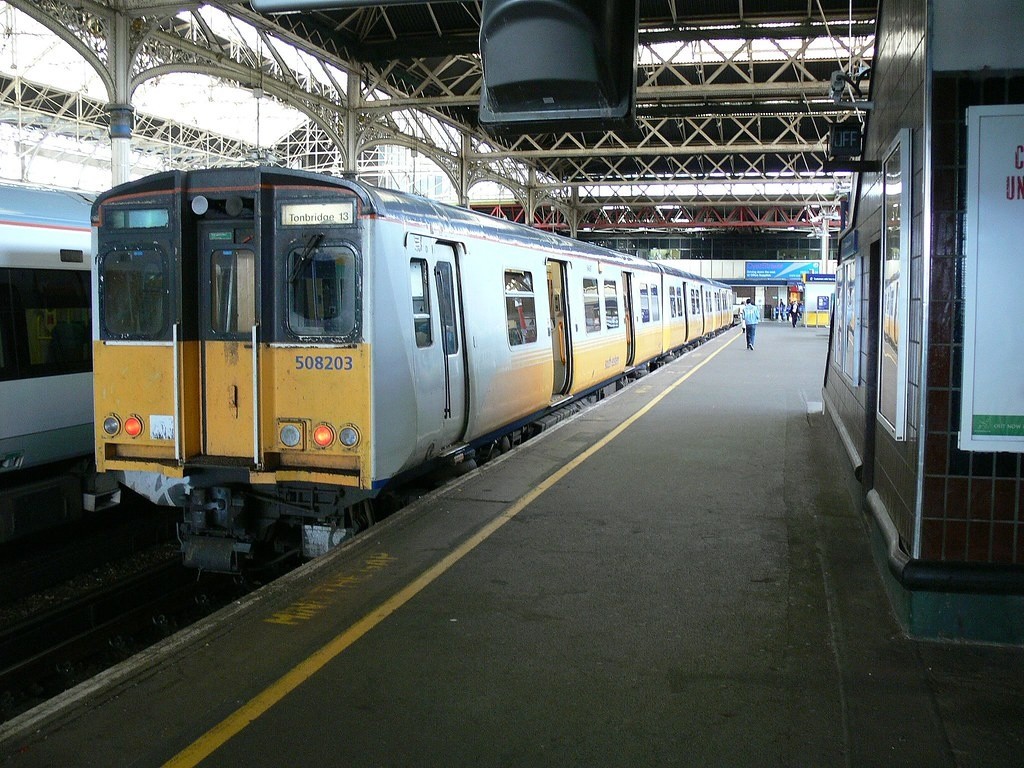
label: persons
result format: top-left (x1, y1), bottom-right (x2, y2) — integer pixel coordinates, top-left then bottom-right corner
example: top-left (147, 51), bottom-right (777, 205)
top-left (780, 299), bottom-right (802, 327)
top-left (738, 299), bottom-right (760, 350)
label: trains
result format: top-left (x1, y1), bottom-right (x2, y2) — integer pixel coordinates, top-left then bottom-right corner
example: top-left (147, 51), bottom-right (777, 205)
top-left (1, 176), bottom-right (136, 542)
top-left (89, 165), bottom-right (733, 573)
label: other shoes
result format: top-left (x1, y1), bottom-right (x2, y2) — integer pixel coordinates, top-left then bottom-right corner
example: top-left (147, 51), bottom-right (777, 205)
top-left (743, 329), bottom-right (745, 333)
top-left (793, 325), bottom-right (796, 328)
top-left (747, 343), bottom-right (754, 350)
